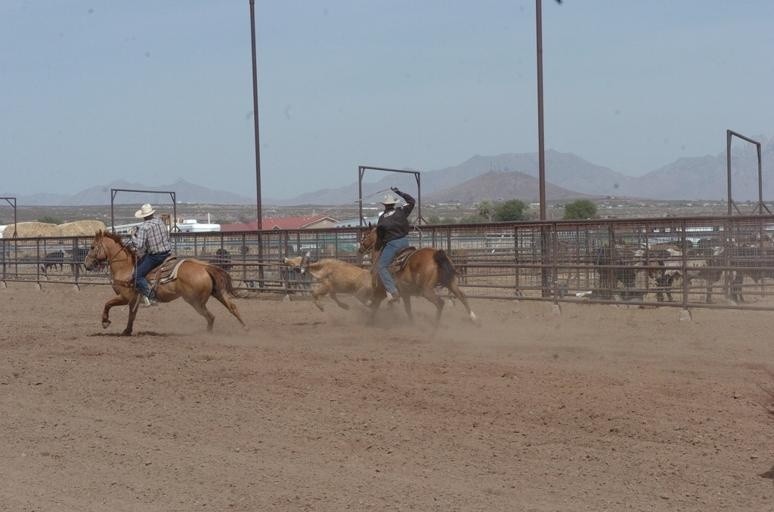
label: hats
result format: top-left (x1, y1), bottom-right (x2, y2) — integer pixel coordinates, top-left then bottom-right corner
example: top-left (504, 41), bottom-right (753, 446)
top-left (379, 194), bottom-right (400, 205)
top-left (134, 204), bottom-right (159, 219)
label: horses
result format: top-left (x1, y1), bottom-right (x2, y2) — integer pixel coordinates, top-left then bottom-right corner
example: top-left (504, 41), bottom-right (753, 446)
top-left (358, 222), bottom-right (478, 326)
top-left (82, 228), bottom-right (251, 336)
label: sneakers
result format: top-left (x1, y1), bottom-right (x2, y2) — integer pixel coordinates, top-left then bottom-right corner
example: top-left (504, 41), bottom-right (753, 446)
top-left (142, 297), bottom-right (156, 308)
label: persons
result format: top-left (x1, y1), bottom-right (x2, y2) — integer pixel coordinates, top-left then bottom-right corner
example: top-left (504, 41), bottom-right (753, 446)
top-left (375, 187), bottom-right (415, 303)
top-left (132, 204), bottom-right (172, 301)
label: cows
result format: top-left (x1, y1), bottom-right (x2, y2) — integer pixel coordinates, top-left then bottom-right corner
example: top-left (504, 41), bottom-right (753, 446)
top-left (41, 250), bottom-right (64, 274)
top-left (281, 248), bottom-right (378, 313)
top-left (595, 237), bottom-right (774, 310)
